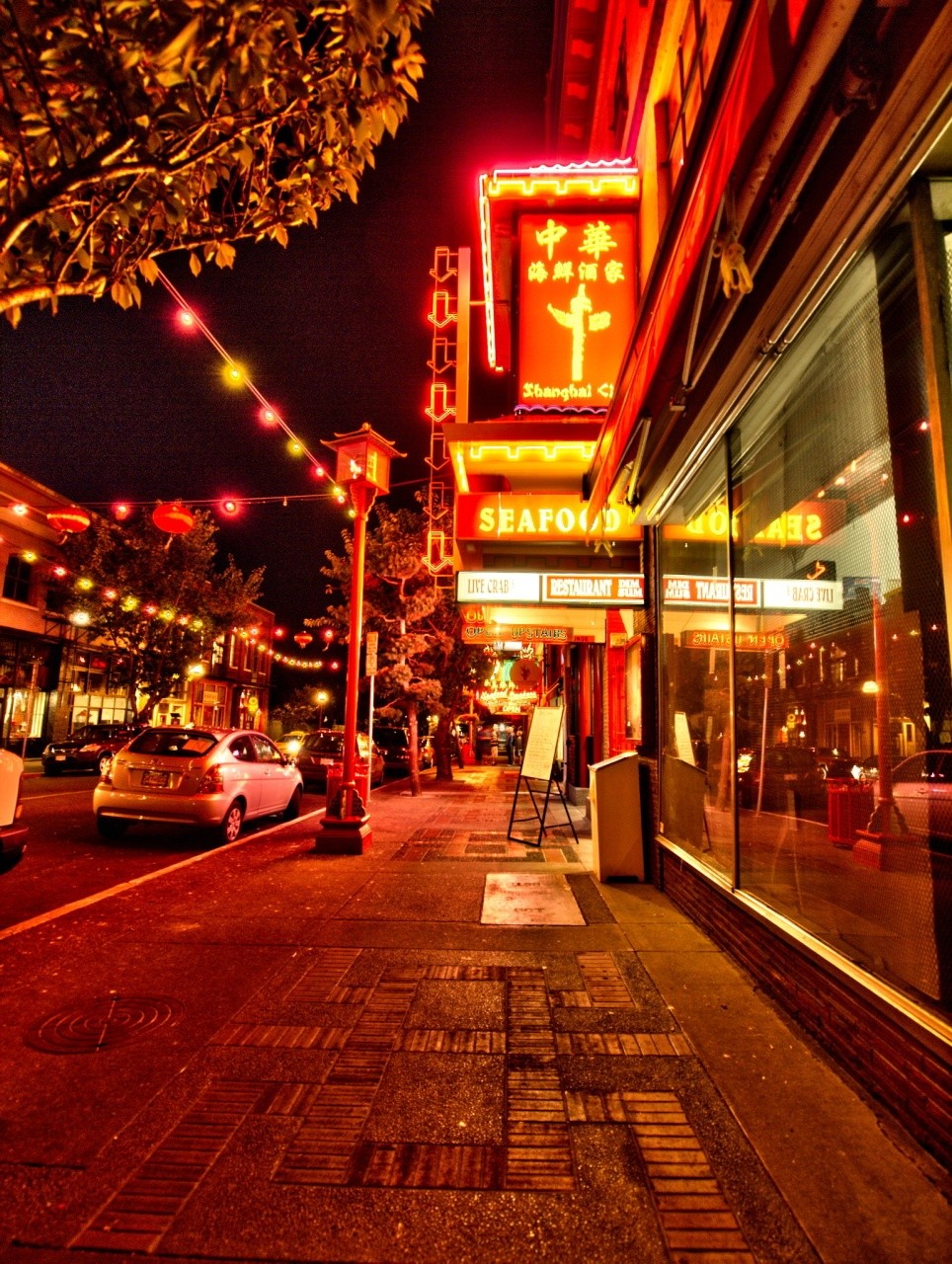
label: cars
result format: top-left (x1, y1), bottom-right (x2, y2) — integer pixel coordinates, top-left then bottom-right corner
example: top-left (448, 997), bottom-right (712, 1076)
top-left (268, 726), bottom-right (457, 790)
top-left (93, 724), bottom-right (304, 845)
top-left (42, 723), bottom-right (161, 775)
top-left (664, 730), bottom-right (952, 846)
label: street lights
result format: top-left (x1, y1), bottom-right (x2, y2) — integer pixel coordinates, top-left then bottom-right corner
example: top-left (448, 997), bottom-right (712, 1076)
top-left (315, 424), bottom-right (407, 853)
top-left (318, 692), bottom-right (326, 730)
top-left (191, 664), bottom-right (204, 725)
top-left (861, 681), bottom-right (878, 769)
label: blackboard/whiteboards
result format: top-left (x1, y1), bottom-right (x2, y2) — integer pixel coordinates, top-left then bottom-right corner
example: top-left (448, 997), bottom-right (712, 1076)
top-left (520, 704), bottom-right (563, 780)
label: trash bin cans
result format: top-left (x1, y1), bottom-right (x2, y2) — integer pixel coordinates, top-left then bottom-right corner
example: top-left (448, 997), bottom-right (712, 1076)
top-left (325, 765), bottom-right (369, 808)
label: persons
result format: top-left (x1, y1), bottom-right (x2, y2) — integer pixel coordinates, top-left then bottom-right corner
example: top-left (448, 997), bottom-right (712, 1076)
top-left (695, 737), bottom-right (707, 784)
top-left (513, 731), bottom-right (523, 766)
top-left (490, 733), bottom-right (499, 766)
top-left (503, 726), bottom-right (515, 767)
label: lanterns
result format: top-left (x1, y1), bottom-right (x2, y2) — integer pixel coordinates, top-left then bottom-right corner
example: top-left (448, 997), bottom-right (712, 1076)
top-left (294, 631), bottom-right (313, 648)
top-left (151, 504), bottom-right (194, 549)
top-left (47, 503), bottom-right (90, 545)
